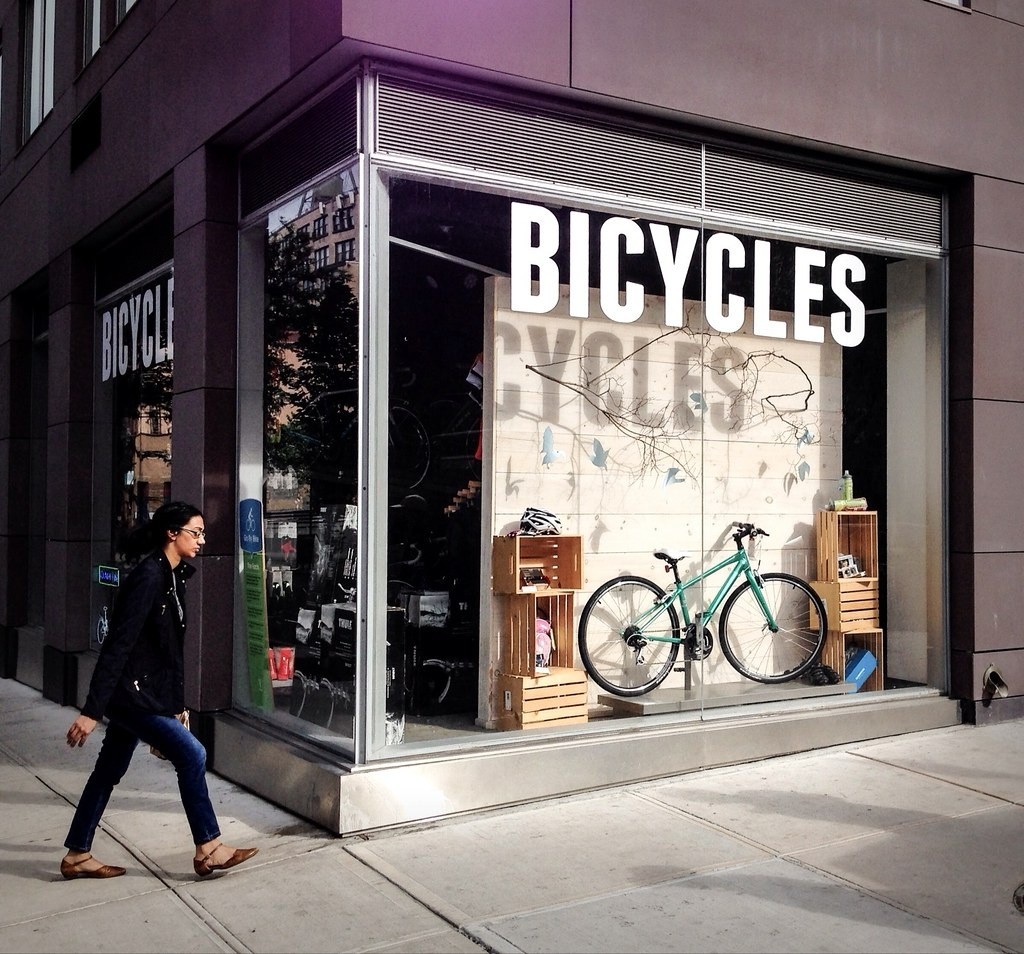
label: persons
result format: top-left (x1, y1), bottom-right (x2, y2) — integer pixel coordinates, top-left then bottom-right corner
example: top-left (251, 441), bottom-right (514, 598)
top-left (61, 501), bottom-right (259, 880)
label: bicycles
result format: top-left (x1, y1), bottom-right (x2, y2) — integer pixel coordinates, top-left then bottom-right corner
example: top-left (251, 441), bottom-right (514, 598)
top-left (576, 519), bottom-right (830, 698)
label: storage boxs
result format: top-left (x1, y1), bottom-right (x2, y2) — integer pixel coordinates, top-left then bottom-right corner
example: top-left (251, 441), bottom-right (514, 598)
top-left (492, 534), bottom-right (584, 595)
top-left (816, 509), bottom-right (880, 581)
top-left (504, 594), bottom-right (575, 674)
top-left (821, 632), bottom-right (884, 691)
top-left (809, 581), bottom-right (880, 632)
top-left (495, 671), bottom-right (590, 730)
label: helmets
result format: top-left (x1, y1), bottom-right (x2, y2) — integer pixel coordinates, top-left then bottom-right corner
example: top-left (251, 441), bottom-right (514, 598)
top-left (520, 508), bottom-right (562, 535)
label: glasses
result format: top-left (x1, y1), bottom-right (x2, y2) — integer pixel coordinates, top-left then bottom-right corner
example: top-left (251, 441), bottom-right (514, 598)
top-left (175, 527), bottom-right (206, 539)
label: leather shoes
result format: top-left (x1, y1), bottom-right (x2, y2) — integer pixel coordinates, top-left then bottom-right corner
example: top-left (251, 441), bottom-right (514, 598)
top-left (61, 855), bottom-right (125, 879)
top-left (193, 843), bottom-right (259, 876)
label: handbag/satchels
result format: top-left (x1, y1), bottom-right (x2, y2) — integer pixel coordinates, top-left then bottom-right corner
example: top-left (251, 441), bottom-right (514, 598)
top-left (148, 712), bottom-right (191, 760)
